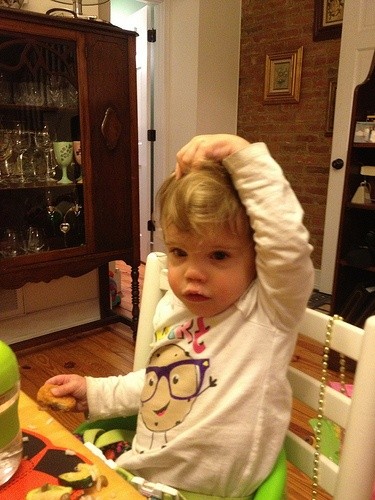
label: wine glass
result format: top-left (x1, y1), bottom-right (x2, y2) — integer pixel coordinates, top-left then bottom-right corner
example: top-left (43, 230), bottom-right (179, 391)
top-left (52, 141), bottom-right (83, 184)
top-left (0, 120), bottom-right (57, 184)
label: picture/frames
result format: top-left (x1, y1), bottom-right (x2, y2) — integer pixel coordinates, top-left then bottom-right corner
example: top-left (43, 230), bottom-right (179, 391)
top-left (325, 77), bottom-right (338, 137)
top-left (261, 44), bottom-right (304, 105)
top-left (312, 0), bottom-right (345, 43)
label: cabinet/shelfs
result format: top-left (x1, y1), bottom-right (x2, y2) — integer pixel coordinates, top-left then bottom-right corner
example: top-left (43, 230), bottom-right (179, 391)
top-left (0, 5), bottom-right (140, 342)
top-left (327, 52), bottom-right (375, 373)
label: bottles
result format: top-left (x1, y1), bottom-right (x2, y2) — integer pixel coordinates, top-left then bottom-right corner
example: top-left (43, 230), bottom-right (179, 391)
top-left (0, 340), bottom-right (23, 485)
top-left (37, 191), bottom-right (85, 249)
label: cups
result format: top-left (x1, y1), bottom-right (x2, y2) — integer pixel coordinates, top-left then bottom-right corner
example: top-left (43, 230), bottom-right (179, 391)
top-left (11, 74), bottom-right (44, 107)
top-left (0, 226), bottom-right (45, 255)
top-left (46, 73), bottom-right (64, 108)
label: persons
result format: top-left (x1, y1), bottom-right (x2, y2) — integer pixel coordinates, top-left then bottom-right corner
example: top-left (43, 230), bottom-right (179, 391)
top-left (36, 132), bottom-right (316, 499)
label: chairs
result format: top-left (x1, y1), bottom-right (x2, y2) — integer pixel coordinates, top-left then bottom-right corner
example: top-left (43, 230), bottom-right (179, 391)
top-left (132, 251), bottom-right (375, 500)
